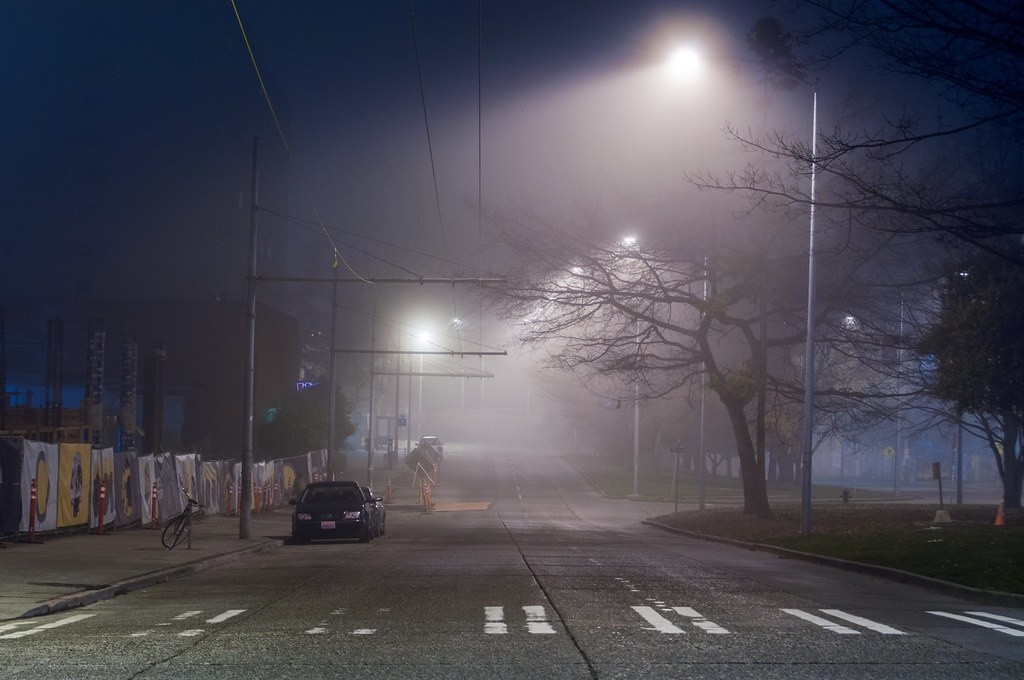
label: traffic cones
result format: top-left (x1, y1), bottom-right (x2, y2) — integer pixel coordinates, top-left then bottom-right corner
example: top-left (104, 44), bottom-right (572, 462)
top-left (993, 497), bottom-right (1007, 526)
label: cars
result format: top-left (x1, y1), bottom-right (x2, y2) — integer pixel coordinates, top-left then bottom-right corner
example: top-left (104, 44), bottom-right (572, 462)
top-left (415, 436), bottom-right (444, 459)
top-left (289, 480), bottom-right (387, 544)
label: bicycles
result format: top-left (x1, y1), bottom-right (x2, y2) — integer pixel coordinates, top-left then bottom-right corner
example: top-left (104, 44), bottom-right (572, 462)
top-left (160, 488), bottom-right (206, 551)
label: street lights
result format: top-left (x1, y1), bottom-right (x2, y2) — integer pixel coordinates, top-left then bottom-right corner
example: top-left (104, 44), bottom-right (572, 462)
top-left (620, 234), bottom-right (710, 510)
top-left (670, 51), bottom-right (822, 539)
top-left (573, 265), bottom-right (643, 500)
